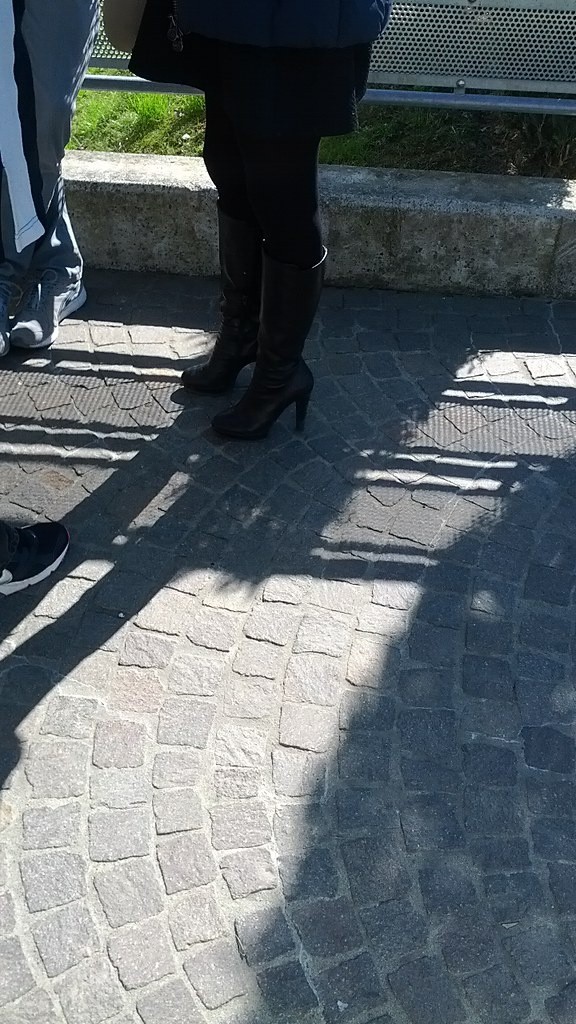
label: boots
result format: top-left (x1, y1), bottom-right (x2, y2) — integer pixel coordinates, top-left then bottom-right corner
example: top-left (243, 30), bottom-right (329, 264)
top-left (181, 199), bottom-right (264, 394)
top-left (211, 244), bottom-right (327, 439)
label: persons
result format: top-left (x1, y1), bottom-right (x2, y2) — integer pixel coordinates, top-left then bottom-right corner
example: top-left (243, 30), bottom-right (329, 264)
top-left (0, 0), bottom-right (100, 357)
top-left (126, 1), bottom-right (388, 445)
top-left (0, 519), bottom-right (70, 599)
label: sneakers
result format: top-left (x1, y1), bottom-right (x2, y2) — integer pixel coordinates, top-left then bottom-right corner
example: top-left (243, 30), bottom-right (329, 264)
top-left (0, 521), bottom-right (70, 600)
top-left (10, 277), bottom-right (87, 348)
top-left (0, 275), bottom-right (35, 356)
